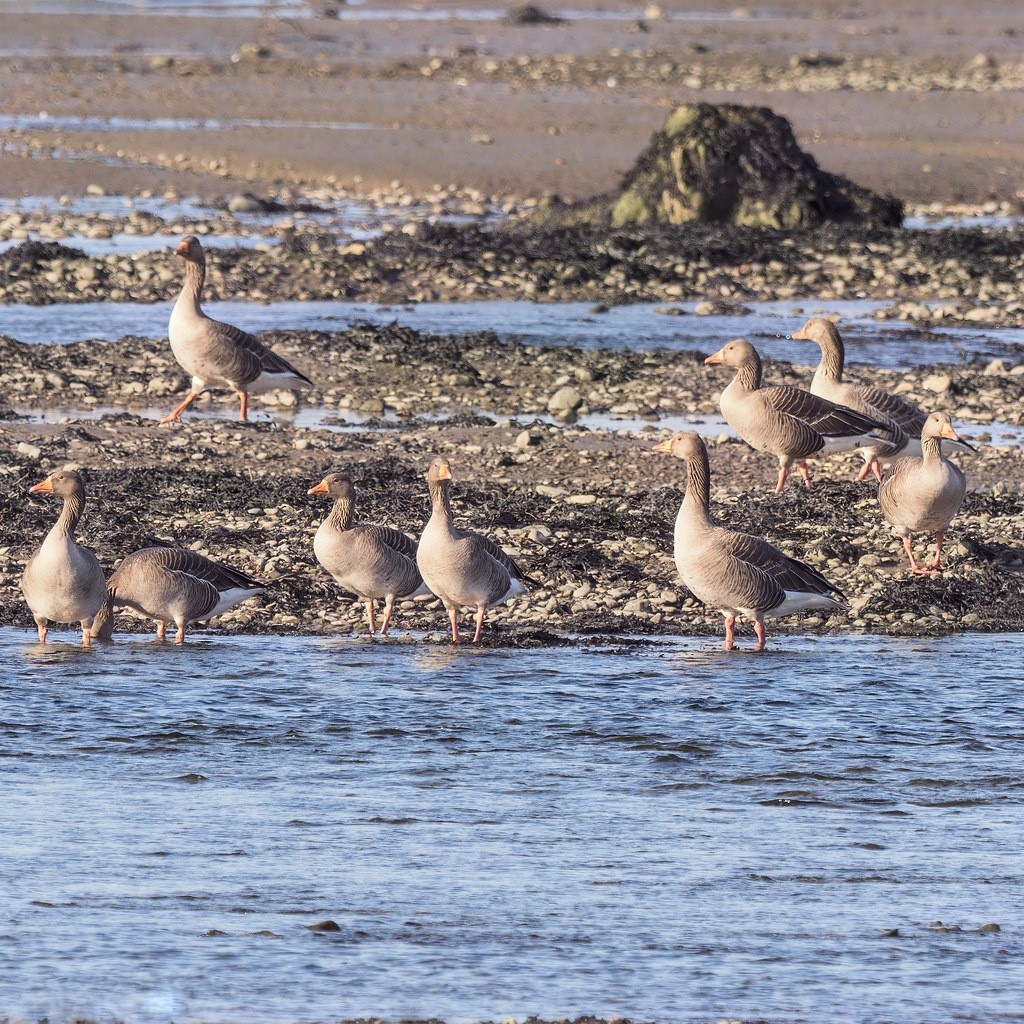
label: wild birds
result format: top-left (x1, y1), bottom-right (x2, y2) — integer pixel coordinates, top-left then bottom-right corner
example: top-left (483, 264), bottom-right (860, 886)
top-left (877, 410), bottom-right (982, 579)
top-left (306, 474), bottom-right (424, 635)
top-left (157, 234), bottom-right (314, 422)
top-left (790, 318), bottom-right (981, 487)
top-left (705, 339), bottom-right (893, 494)
top-left (22, 469), bottom-right (106, 647)
top-left (416, 456), bottom-right (540, 644)
top-left (106, 547), bottom-right (270, 643)
top-left (654, 430), bottom-right (850, 652)
